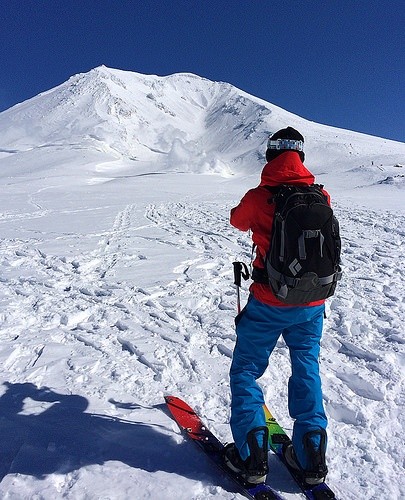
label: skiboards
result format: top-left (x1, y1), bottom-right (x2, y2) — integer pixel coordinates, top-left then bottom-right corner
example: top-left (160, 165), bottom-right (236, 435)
top-left (164, 396), bottom-right (337, 500)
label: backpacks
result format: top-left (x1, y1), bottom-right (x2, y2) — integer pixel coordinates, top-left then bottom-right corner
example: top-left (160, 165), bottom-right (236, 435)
top-left (251, 183), bottom-right (341, 304)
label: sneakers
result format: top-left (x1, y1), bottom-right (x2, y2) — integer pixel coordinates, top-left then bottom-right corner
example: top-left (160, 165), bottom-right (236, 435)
top-left (282, 430), bottom-right (328, 484)
top-left (225, 426), bottom-right (269, 483)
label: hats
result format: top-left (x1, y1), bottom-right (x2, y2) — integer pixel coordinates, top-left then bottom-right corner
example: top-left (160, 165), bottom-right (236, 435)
top-left (266, 126), bottom-right (305, 163)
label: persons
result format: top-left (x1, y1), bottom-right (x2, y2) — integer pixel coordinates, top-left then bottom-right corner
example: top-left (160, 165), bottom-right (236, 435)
top-left (225, 127), bottom-right (331, 486)
